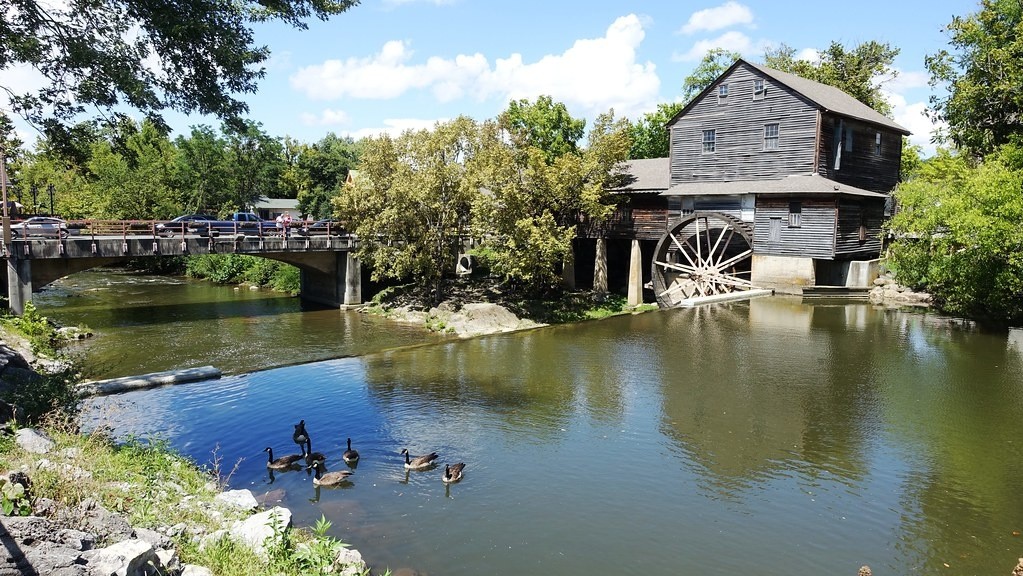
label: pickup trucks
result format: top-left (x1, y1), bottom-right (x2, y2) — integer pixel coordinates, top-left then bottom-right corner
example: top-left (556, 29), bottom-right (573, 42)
top-left (187, 212), bottom-right (282, 237)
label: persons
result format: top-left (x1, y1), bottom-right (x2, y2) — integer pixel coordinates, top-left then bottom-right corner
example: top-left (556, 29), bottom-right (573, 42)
top-left (276, 211), bottom-right (292, 237)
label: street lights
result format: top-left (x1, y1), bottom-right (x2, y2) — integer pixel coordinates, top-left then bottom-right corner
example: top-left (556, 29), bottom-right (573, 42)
top-left (29, 182), bottom-right (40, 214)
top-left (16, 187), bottom-right (23, 214)
top-left (45, 183), bottom-right (56, 215)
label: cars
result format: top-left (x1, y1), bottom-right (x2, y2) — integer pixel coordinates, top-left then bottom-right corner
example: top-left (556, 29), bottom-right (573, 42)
top-left (0, 216), bottom-right (80, 239)
top-left (298, 219), bottom-right (347, 236)
top-left (152, 215), bottom-right (219, 237)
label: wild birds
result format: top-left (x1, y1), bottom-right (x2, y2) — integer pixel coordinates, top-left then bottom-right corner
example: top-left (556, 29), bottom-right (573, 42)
top-left (293, 419), bottom-right (310, 444)
top-left (400, 448), bottom-right (439, 471)
top-left (343, 437), bottom-right (360, 464)
top-left (305, 451), bottom-right (327, 469)
top-left (262, 447), bottom-right (303, 468)
top-left (307, 460), bottom-right (355, 486)
top-left (441, 462), bottom-right (466, 483)
